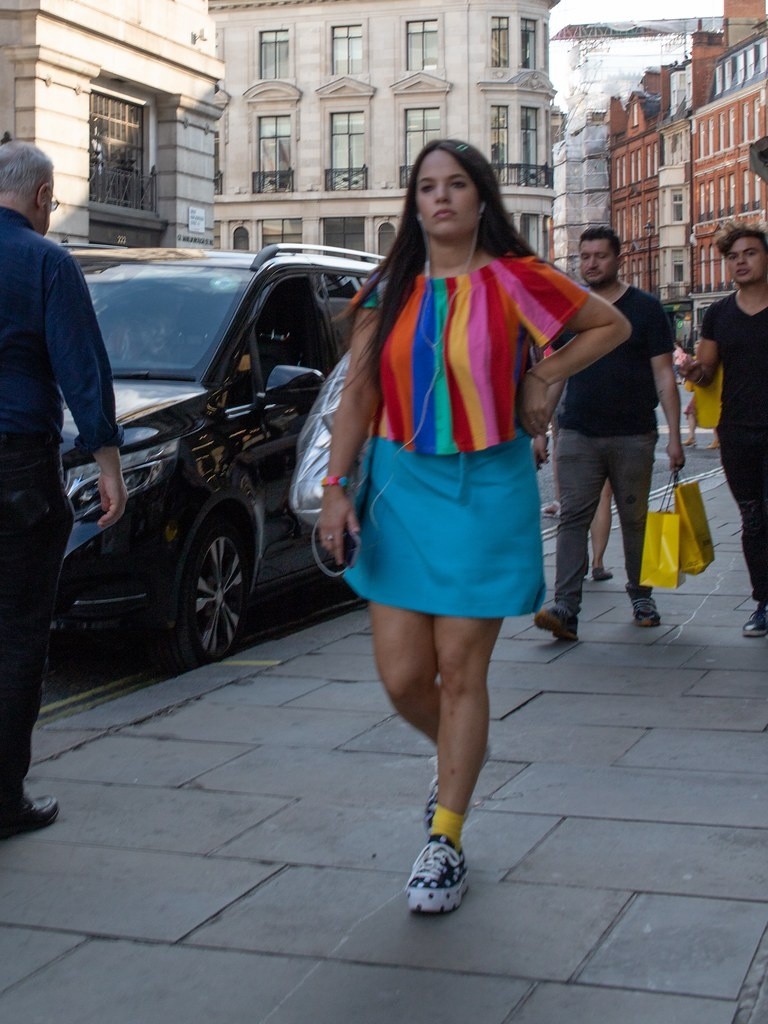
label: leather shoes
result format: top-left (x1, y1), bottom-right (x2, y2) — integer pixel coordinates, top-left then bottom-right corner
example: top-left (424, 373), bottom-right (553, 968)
top-left (0, 793), bottom-right (59, 839)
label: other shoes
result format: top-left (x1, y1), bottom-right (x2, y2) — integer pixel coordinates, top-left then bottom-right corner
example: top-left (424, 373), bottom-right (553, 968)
top-left (591, 566), bottom-right (613, 580)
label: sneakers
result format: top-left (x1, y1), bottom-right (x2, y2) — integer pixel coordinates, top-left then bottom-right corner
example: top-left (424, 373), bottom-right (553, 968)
top-left (407, 833), bottom-right (470, 914)
top-left (741, 602), bottom-right (766, 635)
top-left (631, 595), bottom-right (660, 625)
top-left (424, 742), bottom-right (492, 828)
top-left (534, 604), bottom-right (578, 640)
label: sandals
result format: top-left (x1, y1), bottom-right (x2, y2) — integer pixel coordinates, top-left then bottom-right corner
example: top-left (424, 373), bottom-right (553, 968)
top-left (542, 500), bottom-right (561, 519)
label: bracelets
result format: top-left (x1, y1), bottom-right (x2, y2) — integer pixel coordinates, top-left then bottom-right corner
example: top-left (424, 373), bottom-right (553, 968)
top-left (527, 370), bottom-right (549, 388)
top-left (322, 475), bottom-right (349, 486)
top-left (695, 374), bottom-right (705, 385)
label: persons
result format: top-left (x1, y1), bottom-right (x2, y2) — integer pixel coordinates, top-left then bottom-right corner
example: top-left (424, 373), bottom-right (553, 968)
top-left (0, 140), bottom-right (127, 844)
top-left (318, 140), bottom-right (633, 912)
top-left (525, 225), bottom-right (768, 641)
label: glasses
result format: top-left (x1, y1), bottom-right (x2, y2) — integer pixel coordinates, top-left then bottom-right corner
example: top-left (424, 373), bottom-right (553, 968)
top-left (47, 188), bottom-right (61, 211)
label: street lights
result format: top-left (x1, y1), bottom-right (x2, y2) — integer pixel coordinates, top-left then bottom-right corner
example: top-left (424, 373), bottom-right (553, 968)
top-left (643, 222), bottom-right (655, 295)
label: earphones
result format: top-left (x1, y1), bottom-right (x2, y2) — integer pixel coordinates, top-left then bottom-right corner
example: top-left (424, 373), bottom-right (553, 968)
top-left (417, 214), bottom-right (423, 222)
top-left (479, 200), bottom-right (487, 214)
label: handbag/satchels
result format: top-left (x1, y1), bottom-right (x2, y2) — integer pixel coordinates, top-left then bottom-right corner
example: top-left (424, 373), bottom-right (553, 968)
top-left (639, 480), bottom-right (716, 591)
top-left (288, 348), bottom-right (374, 526)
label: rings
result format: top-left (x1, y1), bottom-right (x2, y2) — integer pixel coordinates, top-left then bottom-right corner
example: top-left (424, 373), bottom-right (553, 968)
top-left (530, 420), bottom-right (537, 425)
top-left (326, 535), bottom-right (335, 540)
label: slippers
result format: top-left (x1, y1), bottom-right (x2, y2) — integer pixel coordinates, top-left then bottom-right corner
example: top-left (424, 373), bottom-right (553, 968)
top-left (683, 440), bottom-right (697, 449)
top-left (707, 441), bottom-right (720, 450)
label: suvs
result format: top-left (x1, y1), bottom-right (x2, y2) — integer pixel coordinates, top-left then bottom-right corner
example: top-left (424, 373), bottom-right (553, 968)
top-left (53, 243), bottom-right (387, 681)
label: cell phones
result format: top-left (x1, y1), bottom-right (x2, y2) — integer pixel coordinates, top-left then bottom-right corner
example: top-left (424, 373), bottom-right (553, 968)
top-left (341, 530), bottom-right (361, 569)
